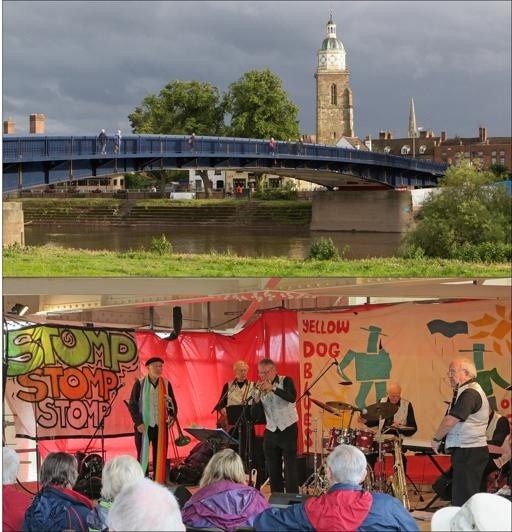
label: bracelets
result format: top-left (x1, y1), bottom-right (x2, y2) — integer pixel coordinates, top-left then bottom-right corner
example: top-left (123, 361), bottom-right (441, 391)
top-left (272, 384), bottom-right (277, 392)
top-left (432, 436), bottom-right (442, 443)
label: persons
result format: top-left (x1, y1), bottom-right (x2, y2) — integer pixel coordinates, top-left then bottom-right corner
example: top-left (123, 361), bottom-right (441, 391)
top-left (129, 357), bottom-right (177, 486)
top-left (365, 381), bottom-right (418, 490)
top-left (431, 356), bottom-right (490, 507)
top-left (251, 359), bottom-right (299, 493)
top-left (216, 359), bottom-right (260, 475)
top-left (480, 403), bottom-right (512, 492)
top-left (1, 445), bottom-right (511, 531)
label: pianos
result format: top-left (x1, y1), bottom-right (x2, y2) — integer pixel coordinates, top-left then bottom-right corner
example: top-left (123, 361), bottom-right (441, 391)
top-left (401, 444), bottom-right (505, 462)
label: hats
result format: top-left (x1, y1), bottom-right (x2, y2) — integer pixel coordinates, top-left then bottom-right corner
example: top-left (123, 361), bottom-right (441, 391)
top-left (145, 357), bottom-right (165, 366)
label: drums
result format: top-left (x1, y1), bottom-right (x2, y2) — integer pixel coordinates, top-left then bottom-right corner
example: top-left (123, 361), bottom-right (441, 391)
top-left (374, 432), bottom-right (404, 453)
top-left (359, 462), bottom-right (376, 491)
top-left (325, 427), bottom-right (352, 451)
top-left (352, 427), bottom-right (374, 454)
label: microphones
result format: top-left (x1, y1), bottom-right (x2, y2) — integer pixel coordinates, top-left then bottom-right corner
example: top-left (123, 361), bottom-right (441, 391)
top-left (112, 382), bottom-right (124, 394)
top-left (246, 395), bottom-right (255, 402)
top-left (355, 408), bottom-right (368, 416)
top-left (230, 376), bottom-right (239, 389)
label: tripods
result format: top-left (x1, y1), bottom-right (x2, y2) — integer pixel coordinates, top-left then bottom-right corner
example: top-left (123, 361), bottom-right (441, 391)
top-left (308, 410), bottom-right (327, 489)
top-left (301, 418), bottom-right (328, 496)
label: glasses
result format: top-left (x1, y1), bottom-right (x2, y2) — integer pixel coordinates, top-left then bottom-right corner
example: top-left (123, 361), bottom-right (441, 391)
top-left (258, 367), bottom-right (274, 374)
top-left (148, 364), bottom-right (163, 368)
top-left (446, 369), bottom-right (465, 375)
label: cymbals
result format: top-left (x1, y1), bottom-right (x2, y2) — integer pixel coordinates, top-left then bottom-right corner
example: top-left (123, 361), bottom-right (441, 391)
top-left (387, 425), bottom-right (416, 431)
top-left (325, 399), bottom-right (353, 410)
top-left (360, 401), bottom-right (398, 421)
top-left (309, 397), bottom-right (342, 417)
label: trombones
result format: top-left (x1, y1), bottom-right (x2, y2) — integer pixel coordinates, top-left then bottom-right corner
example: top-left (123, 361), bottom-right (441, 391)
top-left (162, 395), bottom-right (192, 467)
